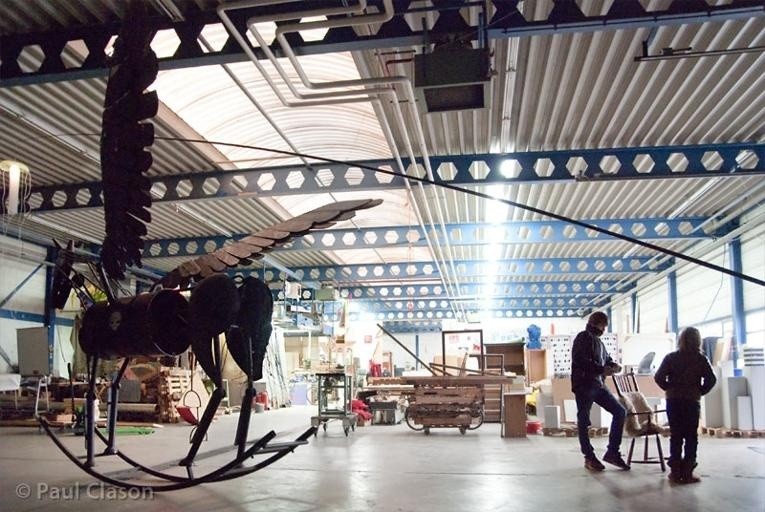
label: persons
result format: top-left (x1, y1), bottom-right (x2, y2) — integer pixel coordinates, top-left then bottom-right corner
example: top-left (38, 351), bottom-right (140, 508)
top-left (570, 311), bottom-right (631, 472)
top-left (654, 327), bottom-right (717, 483)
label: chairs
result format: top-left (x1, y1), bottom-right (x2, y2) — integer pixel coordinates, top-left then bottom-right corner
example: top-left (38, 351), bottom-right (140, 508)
top-left (612, 368), bottom-right (669, 471)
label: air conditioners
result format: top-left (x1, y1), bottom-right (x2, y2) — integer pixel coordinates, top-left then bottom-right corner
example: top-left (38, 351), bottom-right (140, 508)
top-left (742, 344), bottom-right (765, 431)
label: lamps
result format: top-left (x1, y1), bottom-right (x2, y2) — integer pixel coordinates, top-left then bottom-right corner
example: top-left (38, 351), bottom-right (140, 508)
top-left (1, 159), bottom-right (32, 214)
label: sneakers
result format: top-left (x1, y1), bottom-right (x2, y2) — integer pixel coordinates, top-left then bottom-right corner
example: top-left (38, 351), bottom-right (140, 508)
top-left (669, 471), bottom-right (681, 481)
top-left (604, 451), bottom-right (631, 470)
top-left (683, 471), bottom-right (693, 482)
top-left (586, 456), bottom-right (604, 470)
